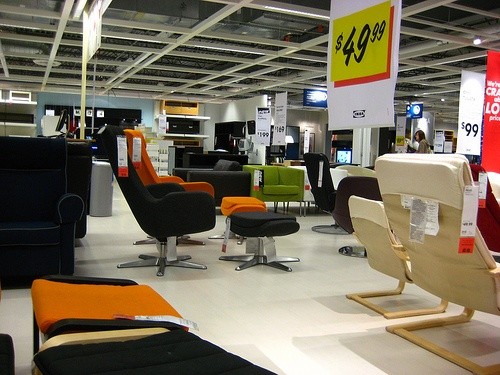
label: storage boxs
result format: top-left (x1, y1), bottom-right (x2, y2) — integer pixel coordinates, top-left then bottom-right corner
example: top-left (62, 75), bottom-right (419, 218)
top-left (167, 118), bottom-right (200, 134)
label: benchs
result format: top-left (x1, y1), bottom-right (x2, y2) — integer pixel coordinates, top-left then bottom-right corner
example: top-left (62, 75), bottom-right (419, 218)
top-left (288, 166), bottom-right (349, 217)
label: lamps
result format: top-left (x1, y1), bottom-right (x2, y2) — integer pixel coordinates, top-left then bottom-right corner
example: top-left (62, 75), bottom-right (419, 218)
top-left (473, 35), bottom-right (483, 45)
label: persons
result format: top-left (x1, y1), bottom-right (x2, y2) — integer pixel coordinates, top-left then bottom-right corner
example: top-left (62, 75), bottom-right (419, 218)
top-left (414, 130), bottom-right (430, 154)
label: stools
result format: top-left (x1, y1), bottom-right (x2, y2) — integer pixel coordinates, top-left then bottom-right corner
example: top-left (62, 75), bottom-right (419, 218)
top-left (29, 327), bottom-right (278, 375)
top-left (30, 273), bottom-right (189, 351)
top-left (218, 212), bottom-right (301, 274)
top-left (207, 196), bottom-right (269, 245)
top-left (0, 331), bottom-right (16, 375)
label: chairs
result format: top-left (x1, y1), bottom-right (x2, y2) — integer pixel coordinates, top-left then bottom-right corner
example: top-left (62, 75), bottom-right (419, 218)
top-left (0, 136), bottom-right (86, 292)
top-left (243, 164), bottom-right (304, 216)
top-left (168, 145), bottom-right (204, 176)
top-left (98, 125), bottom-right (216, 277)
top-left (67, 141), bottom-right (94, 248)
top-left (303, 151), bottom-right (350, 236)
top-left (346, 195), bottom-right (450, 322)
top-left (469, 163), bottom-right (500, 253)
top-left (172, 159), bottom-right (252, 216)
top-left (181, 152), bottom-right (249, 169)
top-left (123, 128), bottom-right (215, 247)
top-left (329, 176), bottom-right (383, 260)
top-left (375, 151), bottom-right (500, 375)
top-left (330, 164), bottom-right (376, 192)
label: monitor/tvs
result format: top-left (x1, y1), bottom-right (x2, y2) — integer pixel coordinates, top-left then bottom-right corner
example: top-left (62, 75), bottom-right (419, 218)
top-left (405, 104), bottom-right (423, 119)
top-left (335, 148), bottom-right (352, 164)
top-left (302, 88), bottom-right (328, 109)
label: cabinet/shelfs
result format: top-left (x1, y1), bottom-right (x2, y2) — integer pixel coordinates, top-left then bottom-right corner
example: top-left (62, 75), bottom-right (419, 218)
top-left (434, 128), bottom-right (454, 154)
top-left (154, 113), bottom-right (211, 139)
top-left (160, 99), bottom-right (200, 115)
